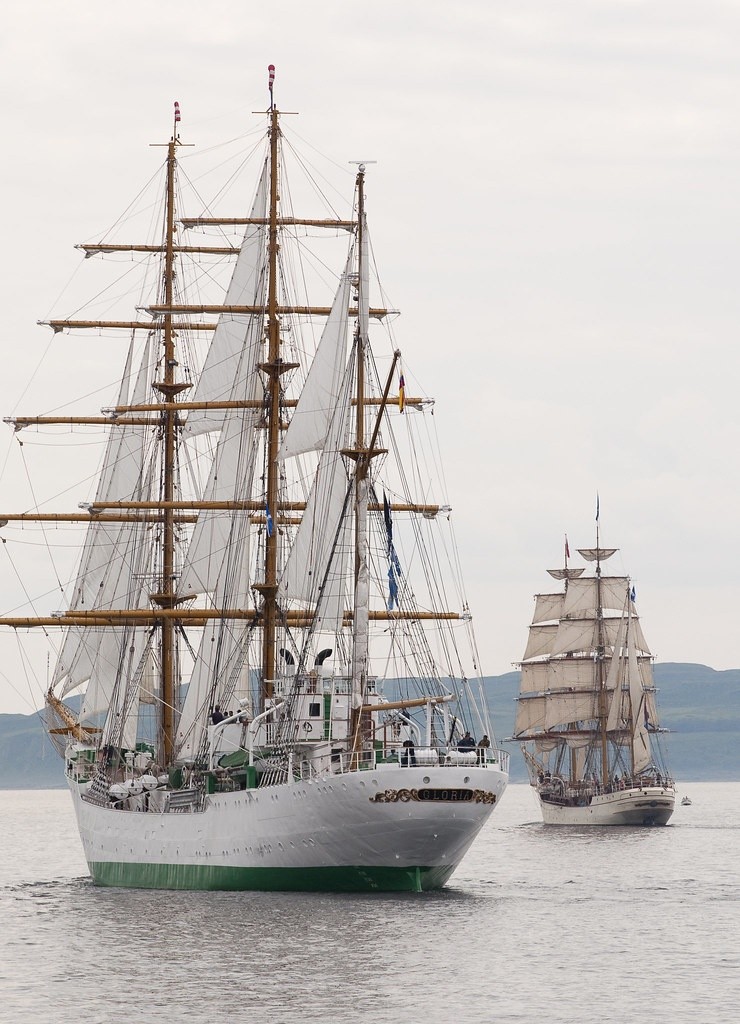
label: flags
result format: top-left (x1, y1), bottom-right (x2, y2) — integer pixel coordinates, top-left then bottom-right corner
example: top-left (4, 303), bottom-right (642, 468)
top-left (630, 586), bottom-right (635, 602)
top-left (265, 504), bottom-right (273, 537)
top-left (399, 374), bottom-right (404, 413)
top-left (566, 543), bottom-right (570, 557)
top-left (383, 494), bottom-right (402, 609)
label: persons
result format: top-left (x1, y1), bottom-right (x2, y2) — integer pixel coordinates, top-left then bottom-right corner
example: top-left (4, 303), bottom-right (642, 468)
top-left (539, 770), bottom-right (550, 783)
top-left (386, 749), bottom-right (398, 762)
top-left (457, 731), bottom-right (476, 752)
top-left (477, 735), bottom-right (491, 749)
top-left (212, 705), bottom-right (243, 724)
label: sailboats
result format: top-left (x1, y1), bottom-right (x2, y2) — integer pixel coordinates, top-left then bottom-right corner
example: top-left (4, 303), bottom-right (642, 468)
top-left (500, 495), bottom-right (678, 829)
top-left (1, 61), bottom-right (514, 894)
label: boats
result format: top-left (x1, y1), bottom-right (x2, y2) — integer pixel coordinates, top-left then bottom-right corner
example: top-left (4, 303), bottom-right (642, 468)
top-left (681, 794), bottom-right (692, 806)
